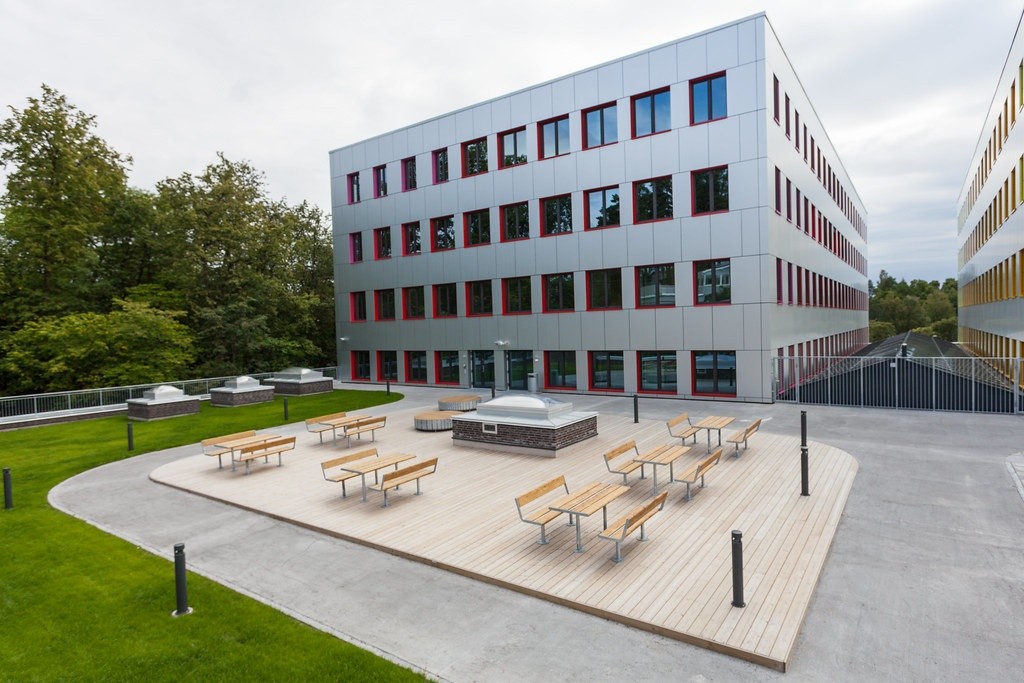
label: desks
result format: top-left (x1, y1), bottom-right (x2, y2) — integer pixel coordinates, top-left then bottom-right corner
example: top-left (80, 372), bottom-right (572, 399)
top-left (692, 415), bottom-right (738, 454)
top-left (633, 443), bottom-right (692, 496)
top-left (340, 452), bottom-right (417, 503)
top-left (214, 433), bottom-right (282, 471)
top-left (318, 415), bottom-right (372, 447)
top-left (549, 481), bottom-right (632, 552)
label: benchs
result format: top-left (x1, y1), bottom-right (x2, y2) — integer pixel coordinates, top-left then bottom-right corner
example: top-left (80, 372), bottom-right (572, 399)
top-left (726, 418), bottom-right (763, 457)
top-left (305, 411), bottom-right (352, 444)
top-left (603, 439), bottom-right (647, 486)
top-left (337, 416), bottom-right (387, 447)
top-left (367, 457), bottom-right (439, 509)
top-left (667, 412), bottom-right (703, 446)
top-left (233, 436), bottom-right (297, 473)
top-left (599, 490), bottom-right (669, 562)
top-left (675, 448), bottom-right (723, 500)
top-left (320, 448), bottom-right (380, 496)
top-left (514, 475), bottom-right (576, 545)
top-left (202, 430), bottom-right (256, 469)
